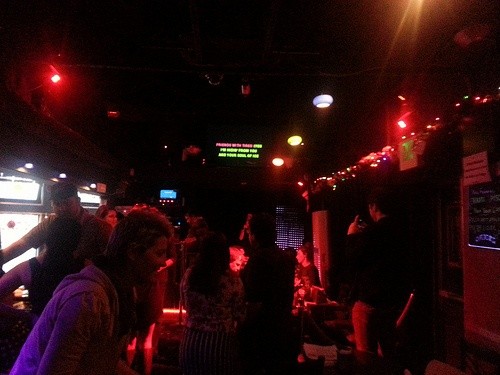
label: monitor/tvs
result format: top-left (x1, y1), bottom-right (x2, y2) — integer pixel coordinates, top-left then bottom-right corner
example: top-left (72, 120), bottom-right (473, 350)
top-left (159, 190), bottom-right (177, 198)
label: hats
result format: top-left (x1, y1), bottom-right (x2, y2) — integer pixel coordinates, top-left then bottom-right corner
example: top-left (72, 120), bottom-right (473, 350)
top-left (51, 182), bottom-right (77, 200)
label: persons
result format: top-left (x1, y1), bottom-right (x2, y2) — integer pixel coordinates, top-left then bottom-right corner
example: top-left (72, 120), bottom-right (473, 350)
top-left (346, 189), bottom-right (407, 352)
top-left (180, 211), bottom-right (319, 375)
top-left (0, 182), bottom-right (175, 375)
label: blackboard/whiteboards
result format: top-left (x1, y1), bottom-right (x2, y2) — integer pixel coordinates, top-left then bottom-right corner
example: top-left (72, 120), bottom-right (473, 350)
top-left (468, 181), bottom-right (500, 250)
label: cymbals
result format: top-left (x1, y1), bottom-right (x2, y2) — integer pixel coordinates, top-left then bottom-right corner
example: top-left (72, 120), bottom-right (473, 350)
top-left (174, 225), bottom-right (184, 230)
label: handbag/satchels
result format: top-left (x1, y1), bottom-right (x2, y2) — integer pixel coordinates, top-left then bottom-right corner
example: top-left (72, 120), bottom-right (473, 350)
top-left (302, 343), bottom-right (339, 367)
